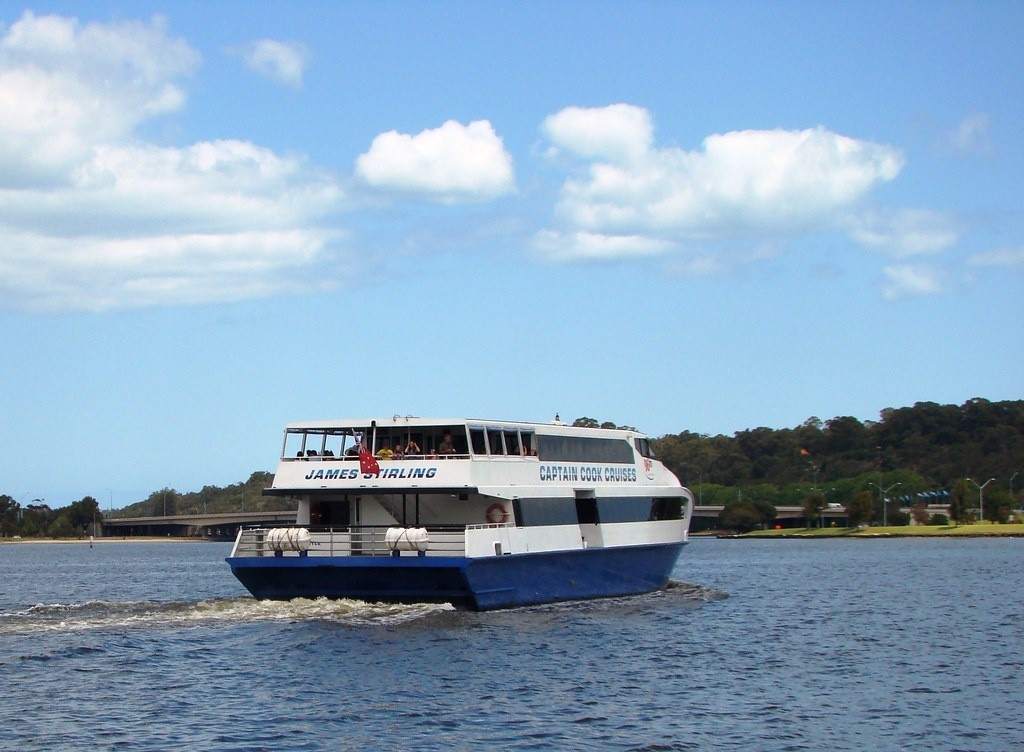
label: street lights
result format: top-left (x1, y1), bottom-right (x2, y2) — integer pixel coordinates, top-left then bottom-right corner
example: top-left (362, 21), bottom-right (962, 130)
top-left (104, 486), bottom-right (113, 511)
top-left (1009, 471), bottom-right (1019, 495)
top-left (796, 485), bottom-right (837, 529)
top-left (868, 482), bottom-right (901, 527)
top-left (964, 477), bottom-right (997, 522)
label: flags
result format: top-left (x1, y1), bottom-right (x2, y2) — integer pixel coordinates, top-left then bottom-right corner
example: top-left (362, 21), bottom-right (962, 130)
top-left (351, 428), bottom-right (380, 478)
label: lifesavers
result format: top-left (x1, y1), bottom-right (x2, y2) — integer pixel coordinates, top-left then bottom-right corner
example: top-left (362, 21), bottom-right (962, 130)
top-left (487, 502), bottom-right (508, 524)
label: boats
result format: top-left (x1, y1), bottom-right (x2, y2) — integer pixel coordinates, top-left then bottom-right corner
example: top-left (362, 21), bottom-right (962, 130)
top-left (224, 412), bottom-right (696, 615)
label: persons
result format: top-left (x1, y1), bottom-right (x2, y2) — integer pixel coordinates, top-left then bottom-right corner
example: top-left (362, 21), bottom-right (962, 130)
top-left (438, 435), bottom-right (456, 460)
top-left (516, 441), bottom-right (527, 456)
top-left (295, 450), bottom-right (333, 461)
top-left (376, 441), bottom-right (422, 460)
